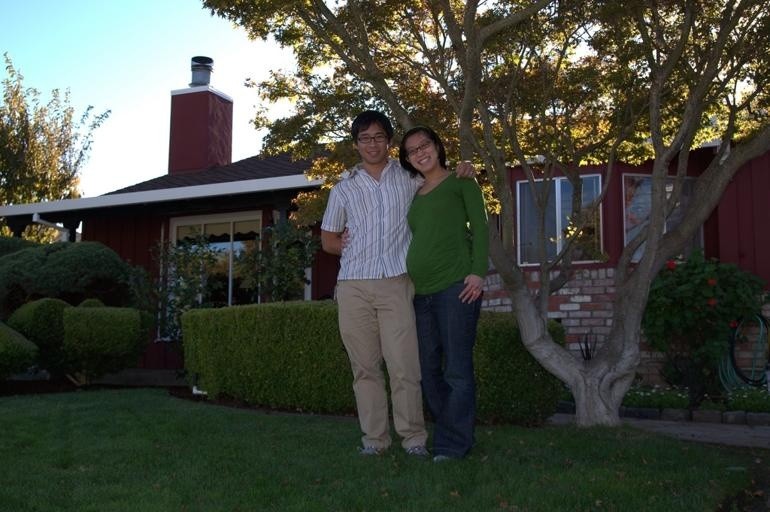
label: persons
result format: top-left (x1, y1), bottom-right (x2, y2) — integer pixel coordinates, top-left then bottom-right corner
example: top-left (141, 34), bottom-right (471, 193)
top-left (319, 107), bottom-right (476, 458)
top-left (339, 122), bottom-right (492, 462)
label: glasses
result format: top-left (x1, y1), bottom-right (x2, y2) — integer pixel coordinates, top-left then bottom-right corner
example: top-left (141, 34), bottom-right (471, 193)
top-left (358, 133), bottom-right (386, 143)
top-left (406, 141), bottom-right (433, 157)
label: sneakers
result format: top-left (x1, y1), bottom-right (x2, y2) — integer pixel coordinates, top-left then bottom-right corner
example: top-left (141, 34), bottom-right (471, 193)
top-left (434, 455), bottom-right (451, 463)
top-left (360, 446), bottom-right (382, 457)
top-left (407, 444), bottom-right (430, 458)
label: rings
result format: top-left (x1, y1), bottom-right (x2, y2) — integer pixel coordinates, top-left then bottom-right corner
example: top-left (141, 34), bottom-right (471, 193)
top-left (474, 291), bottom-right (477, 294)
